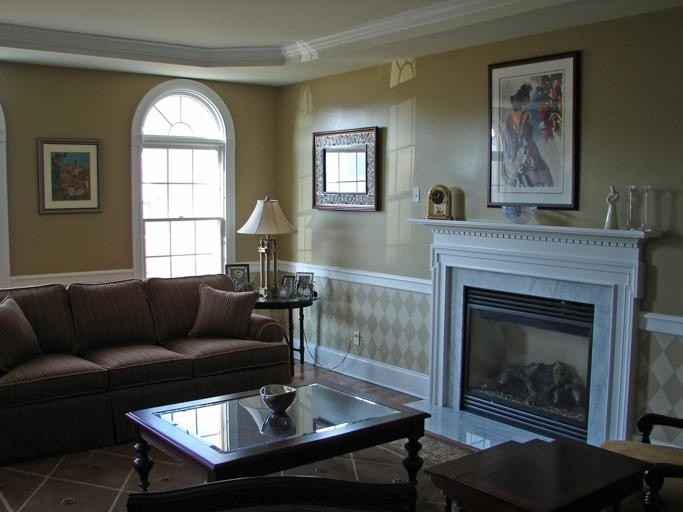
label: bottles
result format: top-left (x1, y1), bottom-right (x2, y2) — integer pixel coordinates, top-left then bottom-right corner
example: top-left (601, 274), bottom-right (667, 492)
top-left (643, 184), bottom-right (657, 228)
top-left (625, 183), bottom-right (642, 229)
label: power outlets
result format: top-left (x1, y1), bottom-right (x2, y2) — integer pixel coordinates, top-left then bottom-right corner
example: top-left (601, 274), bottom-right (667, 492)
top-left (354, 332), bottom-right (359, 345)
top-left (413, 187), bottom-right (419, 204)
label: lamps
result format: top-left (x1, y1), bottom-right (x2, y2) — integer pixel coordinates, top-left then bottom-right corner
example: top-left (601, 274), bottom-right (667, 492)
top-left (236, 196), bottom-right (298, 297)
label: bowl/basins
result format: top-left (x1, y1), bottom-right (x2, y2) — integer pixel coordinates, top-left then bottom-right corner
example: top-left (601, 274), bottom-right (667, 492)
top-left (260, 384), bottom-right (297, 412)
top-left (502, 205), bottom-right (537, 224)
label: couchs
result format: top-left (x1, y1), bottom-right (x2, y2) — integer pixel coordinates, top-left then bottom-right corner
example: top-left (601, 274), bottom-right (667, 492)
top-left (0, 274), bottom-right (291, 466)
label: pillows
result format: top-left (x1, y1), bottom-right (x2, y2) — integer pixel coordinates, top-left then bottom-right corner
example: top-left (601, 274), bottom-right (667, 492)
top-left (0, 274), bottom-right (257, 376)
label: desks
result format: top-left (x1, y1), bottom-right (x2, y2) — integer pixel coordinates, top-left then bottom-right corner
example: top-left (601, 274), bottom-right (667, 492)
top-left (426, 437), bottom-right (642, 512)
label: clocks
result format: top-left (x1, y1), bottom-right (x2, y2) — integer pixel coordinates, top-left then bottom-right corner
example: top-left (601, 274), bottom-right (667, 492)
top-left (426, 183), bottom-right (452, 220)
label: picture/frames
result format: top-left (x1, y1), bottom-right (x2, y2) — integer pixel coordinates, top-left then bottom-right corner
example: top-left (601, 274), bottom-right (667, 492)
top-left (226, 264), bottom-right (249, 292)
top-left (487, 50), bottom-right (581, 211)
top-left (296, 273), bottom-right (313, 300)
top-left (38, 137), bottom-right (104, 215)
top-left (281, 275), bottom-right (295, 288)
top-left (313, 126), bottom-right (379, 211)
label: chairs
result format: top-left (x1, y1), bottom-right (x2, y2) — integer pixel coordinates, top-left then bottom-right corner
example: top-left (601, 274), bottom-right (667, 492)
top-left (601, 413), bottom-right (683, 512)
top-left (127, 475), bottom-right (418, 512)
